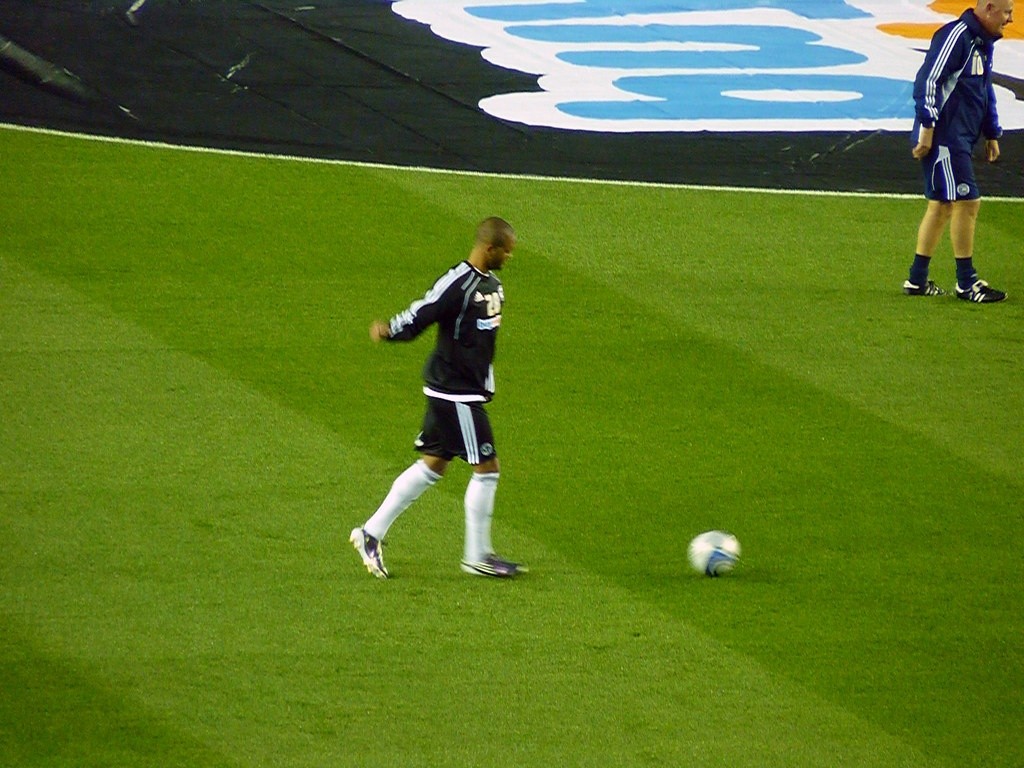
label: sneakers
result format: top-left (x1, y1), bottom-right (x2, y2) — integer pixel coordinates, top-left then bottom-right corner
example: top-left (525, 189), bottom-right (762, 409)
top-left (903, 278), bottom-right (946, 296)
top-left (459, 555), bottom-right (517, 578)
top-left (350, 527), bottom-right (390, 580)
top-left (956, 279), bottom-right (1008, 303)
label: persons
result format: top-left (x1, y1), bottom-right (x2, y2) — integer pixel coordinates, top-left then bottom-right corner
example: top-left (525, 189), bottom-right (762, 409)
top-left (903, 0), bottom-right (1013, 303)
top-left (349, 217), bottom-right (519, 579)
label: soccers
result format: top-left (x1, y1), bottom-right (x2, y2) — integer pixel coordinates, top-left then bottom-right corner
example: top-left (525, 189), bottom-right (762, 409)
top-left (686, 530), bottom-right (742, 577)
